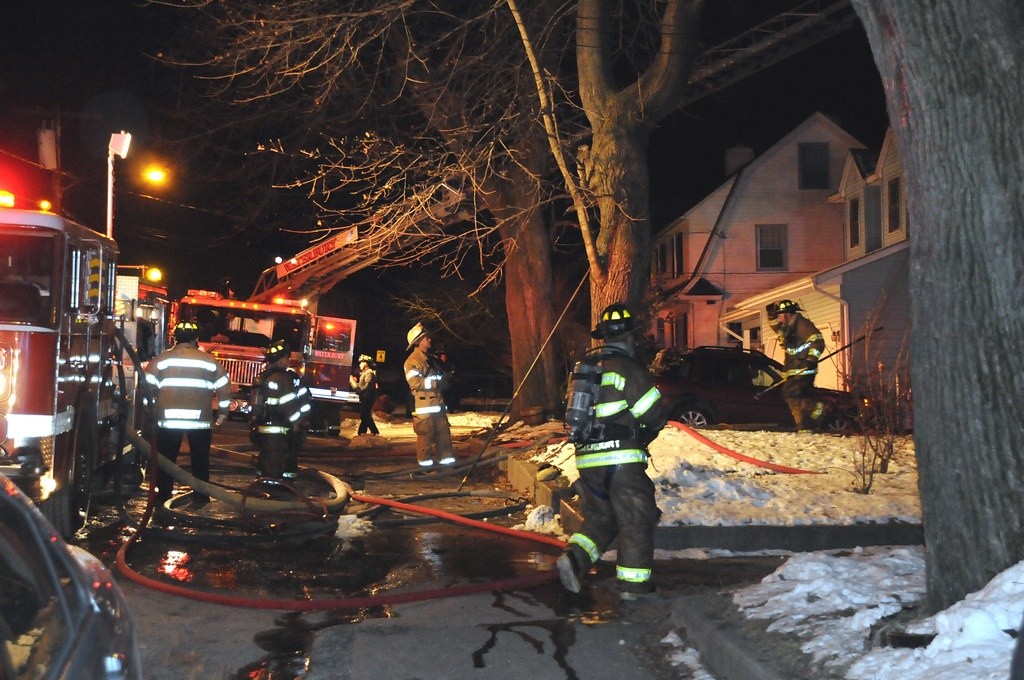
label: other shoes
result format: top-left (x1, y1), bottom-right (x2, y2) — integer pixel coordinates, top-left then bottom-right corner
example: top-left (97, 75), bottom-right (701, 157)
top-left (617, 579), bottom-right (657, 601)
top-left (556, 550), bottom-right (580, 594)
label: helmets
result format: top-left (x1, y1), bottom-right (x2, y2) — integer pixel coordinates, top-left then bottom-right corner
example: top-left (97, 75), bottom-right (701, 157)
top-left (776, 299), bottom-right (805, 313)
top-left (405, 321), bottom-right (430, 352)
top-left (590, 302), bottom-right (644, 340)
top-left (265, 336), bottom-right (293, 365)
top-left (171, 321), bottom-right (199, 337)
top-left (358, 353), bottom-right (374, 362)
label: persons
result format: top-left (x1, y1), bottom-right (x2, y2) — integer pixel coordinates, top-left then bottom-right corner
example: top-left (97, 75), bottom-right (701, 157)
top-left (404, 322), bottom-right (456, 467)
top-left (557, 303), bottom-right (668, 600)
top-left (144, 319), bottom-right (231, 503)
top-left (766, 300), bottom-right (833, 434)
top-left (256, 338), bottom-right (311, 479)
top-left (349, 355), bottom-right (380, 436)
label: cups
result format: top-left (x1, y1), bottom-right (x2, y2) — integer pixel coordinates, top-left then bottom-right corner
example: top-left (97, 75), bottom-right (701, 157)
top-left (331, 387), bottom-right (337, 397)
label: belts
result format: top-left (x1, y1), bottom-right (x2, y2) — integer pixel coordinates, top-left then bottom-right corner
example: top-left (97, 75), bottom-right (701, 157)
top-left (420, 396), bottom-right (436, 400)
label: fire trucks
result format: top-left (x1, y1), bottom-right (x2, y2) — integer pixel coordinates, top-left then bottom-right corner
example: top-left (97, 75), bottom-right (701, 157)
top-left (1, 1), bottom-right (857, 543)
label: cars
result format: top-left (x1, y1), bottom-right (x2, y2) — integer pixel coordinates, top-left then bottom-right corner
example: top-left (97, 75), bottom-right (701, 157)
top-left (0, 471), bottom-right (144, 680)
top-left (648, 343), bottom-right (876, 436)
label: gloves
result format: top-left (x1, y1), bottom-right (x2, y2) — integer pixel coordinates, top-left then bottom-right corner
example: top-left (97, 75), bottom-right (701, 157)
top-left (803, 356), bottom-right (818, 370)
top-left (765, 303), bottom-right (780, 316)
